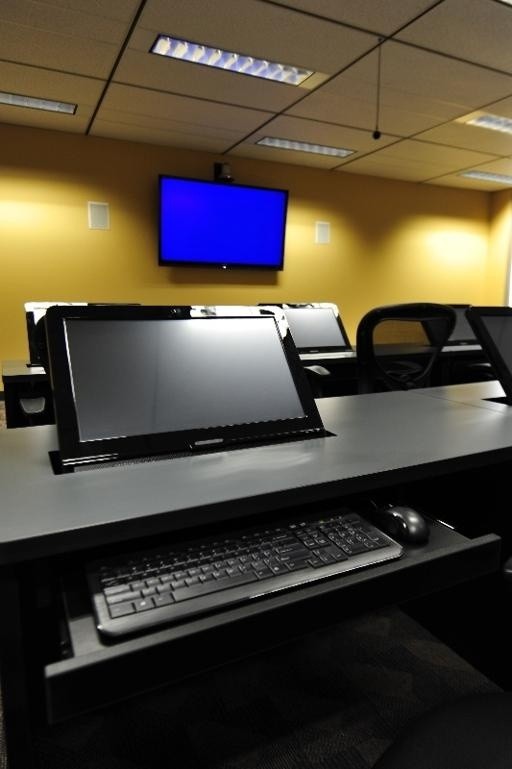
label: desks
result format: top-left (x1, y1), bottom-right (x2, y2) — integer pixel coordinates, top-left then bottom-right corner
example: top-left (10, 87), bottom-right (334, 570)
top-left (0, 304), bottom-right (512, 769)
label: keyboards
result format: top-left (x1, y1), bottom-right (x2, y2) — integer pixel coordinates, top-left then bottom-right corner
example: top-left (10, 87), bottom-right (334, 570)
top-left (87, 508), bottom-right (402, 643)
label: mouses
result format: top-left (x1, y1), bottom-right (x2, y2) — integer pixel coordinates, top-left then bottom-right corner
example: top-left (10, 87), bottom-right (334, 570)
top-left (364, 499), bottom-right (432, 545)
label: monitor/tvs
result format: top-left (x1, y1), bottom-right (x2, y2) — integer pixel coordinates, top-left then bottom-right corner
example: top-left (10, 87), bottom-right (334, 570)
top-left (42, 306), bottom-right (324, 468)
top-left (464, 302), bottom-right (512, 398)
top-left (155, 175), bottom-right (289, 269)
top-left (431, 304), bottom-right (477, 345)
top-left (258, 303), bottom-right (354, 352)
top-left (25, 303), bottom-right (143, 366)
